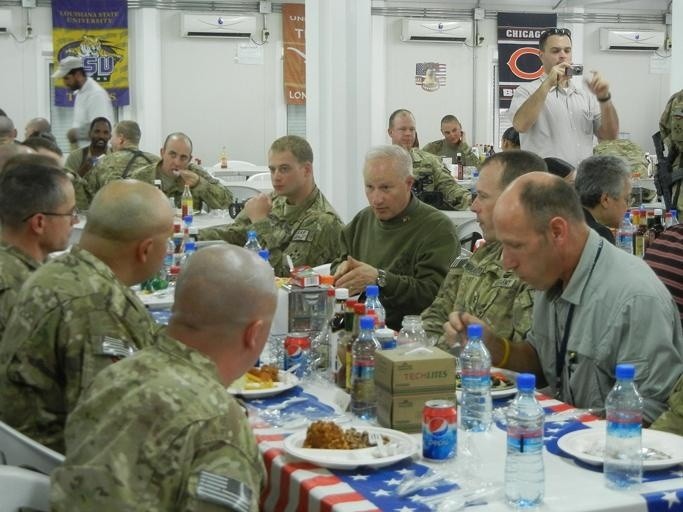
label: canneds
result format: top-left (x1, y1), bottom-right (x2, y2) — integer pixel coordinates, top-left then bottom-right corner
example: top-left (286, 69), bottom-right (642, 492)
top-left (283, 333), bottom-right (311, 369)
top-left (422, 398), bottom-right (457, 461)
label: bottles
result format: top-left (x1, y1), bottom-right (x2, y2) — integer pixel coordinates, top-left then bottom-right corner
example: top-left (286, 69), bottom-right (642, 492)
top-left (502, 374), bottom-right (545, 507)
top-left (456, 153), bottom-right (463, 180)
top-left (149, 177), bottom-right (198, 286)
top-left (329, 286), bottom-right (392, 394)
top-left (396, 314), bottom-right (426, 353)
top-left (219, 145), bottom-right (226, 169)
top-left (616, 208), bottom-right (678, 256)
top-left (350, 319), bottom-right (381, 419)
top-left (603, 364), bottom-right (644, 494)
top-left (459, 325), bottom-right (492, 431)
top-left (630, 172), bottom-right (643, 207)
top-left (470, 173), bottom-right (477, 192)
top-left (243, 229), bottom-right (258, 256)
top-left (470, 144), bottom-right (495, 162)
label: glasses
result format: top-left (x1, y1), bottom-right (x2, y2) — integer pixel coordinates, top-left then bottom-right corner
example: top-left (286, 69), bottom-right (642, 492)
top-left (22, 205), bottom-right (78, 223)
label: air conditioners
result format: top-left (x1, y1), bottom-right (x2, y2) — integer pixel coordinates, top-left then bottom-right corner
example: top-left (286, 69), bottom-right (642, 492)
top-left (598, 28), bottom-right (665, 53)
top-left (401, 20), bottom-right (473, 43)
top-left (182, 13), bottom-right (257, 38)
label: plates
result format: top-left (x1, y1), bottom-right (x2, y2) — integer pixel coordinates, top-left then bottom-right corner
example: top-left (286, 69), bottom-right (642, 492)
top-left (556, 425), bottom-right (682, 470)
top-left (455, 366), bottom-right (523, 399)
top-left (281, 426), bottom-right (417, 470)
top-left (139, 295), bottom-right (175, 309)
top-left (228, 365), bottom-right (299, 401)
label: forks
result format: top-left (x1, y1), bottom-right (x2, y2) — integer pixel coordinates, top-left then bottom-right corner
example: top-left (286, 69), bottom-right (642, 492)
top-left (368, 431), bottom-right (387, 457)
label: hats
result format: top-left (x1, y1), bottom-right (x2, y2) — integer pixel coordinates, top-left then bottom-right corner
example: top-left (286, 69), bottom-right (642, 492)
top-left (52, 57), bottom-right (85, 80)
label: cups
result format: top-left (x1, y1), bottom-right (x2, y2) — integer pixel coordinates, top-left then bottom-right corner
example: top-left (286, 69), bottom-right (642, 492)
top-left (442, 156), bottom-right (457, 179)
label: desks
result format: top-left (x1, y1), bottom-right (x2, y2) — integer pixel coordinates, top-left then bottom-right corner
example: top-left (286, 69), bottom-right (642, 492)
top-left (0, 158), bottom-right (682, 512)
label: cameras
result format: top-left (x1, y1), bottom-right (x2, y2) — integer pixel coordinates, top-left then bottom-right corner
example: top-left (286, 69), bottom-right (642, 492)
top-left (566, 65), bottom-right (583, 75)
